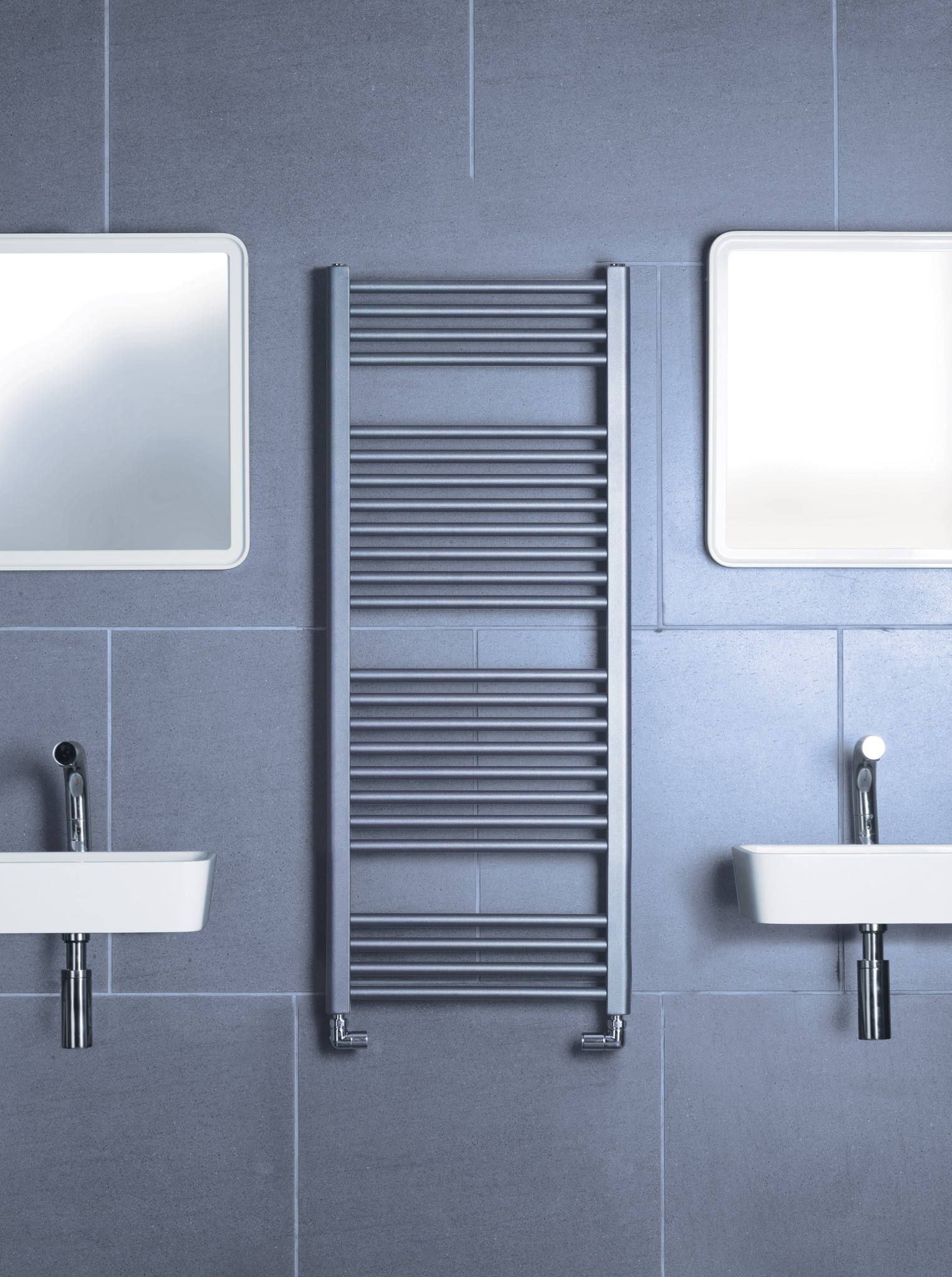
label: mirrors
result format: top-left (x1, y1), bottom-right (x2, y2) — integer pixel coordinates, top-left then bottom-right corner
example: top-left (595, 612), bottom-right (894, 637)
top-left (704, 231), bottom-right (952, 569)
top-left (1, 231), bottom-right (251, 574)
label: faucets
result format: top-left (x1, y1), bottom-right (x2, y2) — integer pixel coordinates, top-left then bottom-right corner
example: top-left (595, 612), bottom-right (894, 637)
top-left (852, 735), bottom-right (884, 845)
top-left (50, 741), bottom-right (91, 852)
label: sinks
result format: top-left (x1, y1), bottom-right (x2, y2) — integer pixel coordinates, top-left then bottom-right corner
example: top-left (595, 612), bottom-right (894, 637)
top-left (730, 842), bottom-right (952, 927)
top-left (0, 850), bottom-right (218, 935)
